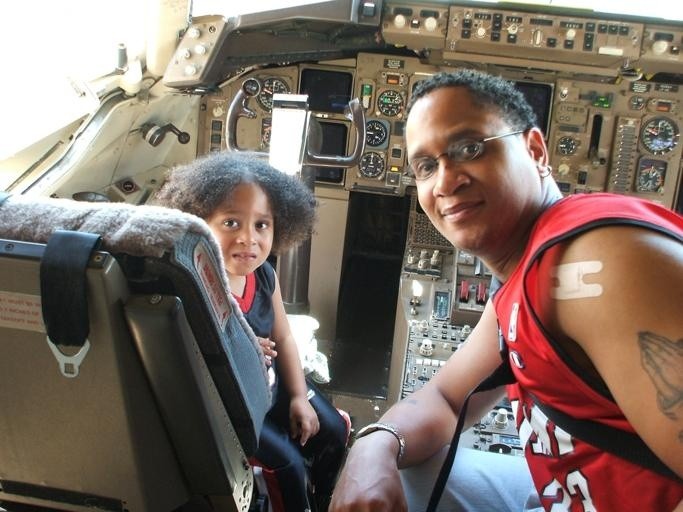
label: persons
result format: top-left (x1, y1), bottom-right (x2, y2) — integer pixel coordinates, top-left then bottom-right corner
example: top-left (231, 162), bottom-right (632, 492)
top-left (324, 70), bottom-right (682, 511)
top-left (155, 149), bottom-right (348, 512)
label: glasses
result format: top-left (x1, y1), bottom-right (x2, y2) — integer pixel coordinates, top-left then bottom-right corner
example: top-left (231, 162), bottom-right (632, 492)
top-left (402, 131), bottom-right (524, 180)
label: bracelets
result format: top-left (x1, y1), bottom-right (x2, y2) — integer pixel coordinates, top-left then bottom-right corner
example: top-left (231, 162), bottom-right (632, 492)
top-left (353, 422), bottom-right (409, 468)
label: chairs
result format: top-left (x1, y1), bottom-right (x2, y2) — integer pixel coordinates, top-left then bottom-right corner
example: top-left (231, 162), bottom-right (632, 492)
top-left (0, 191), bottom-right (333, 511)
